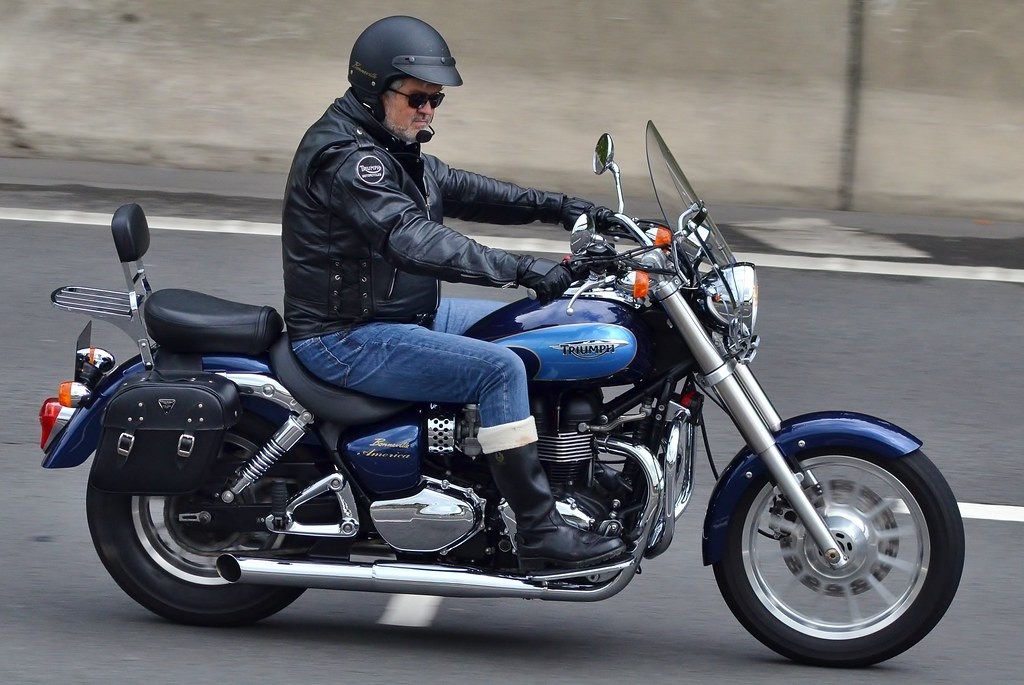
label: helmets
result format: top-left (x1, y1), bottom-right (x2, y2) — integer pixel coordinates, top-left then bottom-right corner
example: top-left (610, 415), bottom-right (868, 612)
top-left (347, 15), bottom-right (463, 123)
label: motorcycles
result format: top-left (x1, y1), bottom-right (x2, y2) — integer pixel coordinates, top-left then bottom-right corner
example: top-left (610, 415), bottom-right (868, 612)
top-left (36, 121), bottom-right (967, 668)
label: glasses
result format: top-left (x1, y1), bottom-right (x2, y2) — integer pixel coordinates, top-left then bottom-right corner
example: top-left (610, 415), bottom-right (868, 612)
top-left (386, 86), bottom-right (447, 110)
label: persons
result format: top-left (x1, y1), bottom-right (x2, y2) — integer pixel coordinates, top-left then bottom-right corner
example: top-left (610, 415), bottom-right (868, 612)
top-left (282, 15), bottom-right (626, 573)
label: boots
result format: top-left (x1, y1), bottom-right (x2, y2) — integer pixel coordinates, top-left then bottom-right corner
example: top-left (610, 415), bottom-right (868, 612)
top-left (477, 416), bottom-right (628, 573)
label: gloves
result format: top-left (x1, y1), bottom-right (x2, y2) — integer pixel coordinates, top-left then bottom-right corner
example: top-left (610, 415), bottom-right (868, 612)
top-left (517, 253), bottom-right (574, 306)
top-left (563, 195), bottom-right (616, 234)
